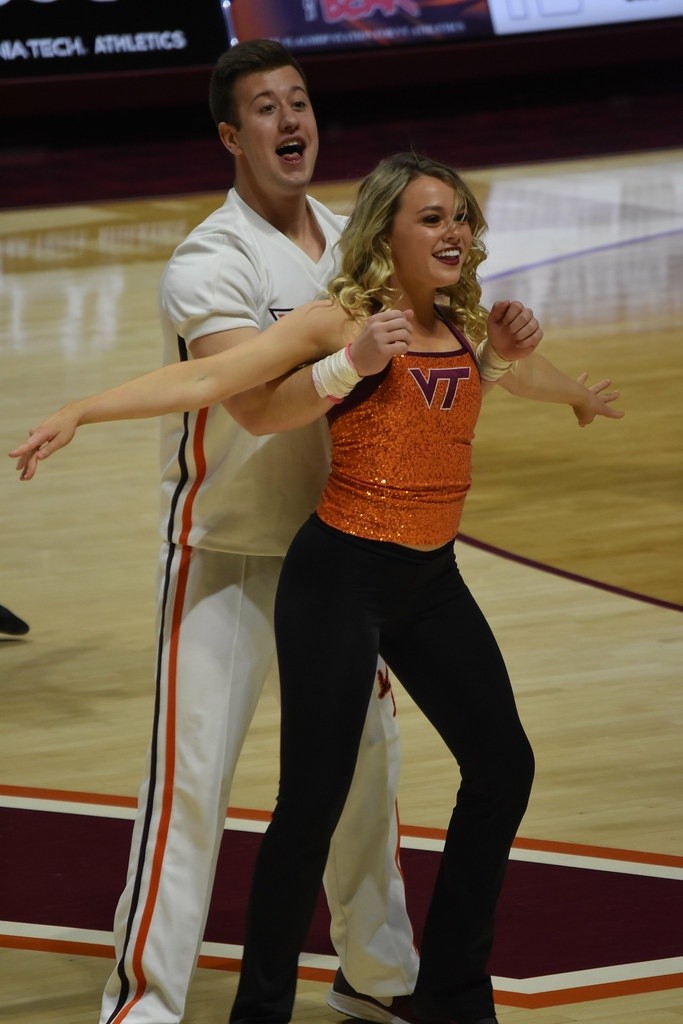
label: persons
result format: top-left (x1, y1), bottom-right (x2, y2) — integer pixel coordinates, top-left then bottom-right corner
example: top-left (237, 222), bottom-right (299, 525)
top-left (9, 152), bottom-right (626, 1024)
top-left (100, 39), bottom-right (421, 1024)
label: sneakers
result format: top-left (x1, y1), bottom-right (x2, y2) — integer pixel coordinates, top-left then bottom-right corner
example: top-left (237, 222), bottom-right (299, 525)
top-left (329, 966), bottom-right (397, 1023)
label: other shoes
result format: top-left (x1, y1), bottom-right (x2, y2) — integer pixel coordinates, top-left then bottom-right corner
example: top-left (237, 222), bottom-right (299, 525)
top-left (0, 604), bottom-right (30, 636)
